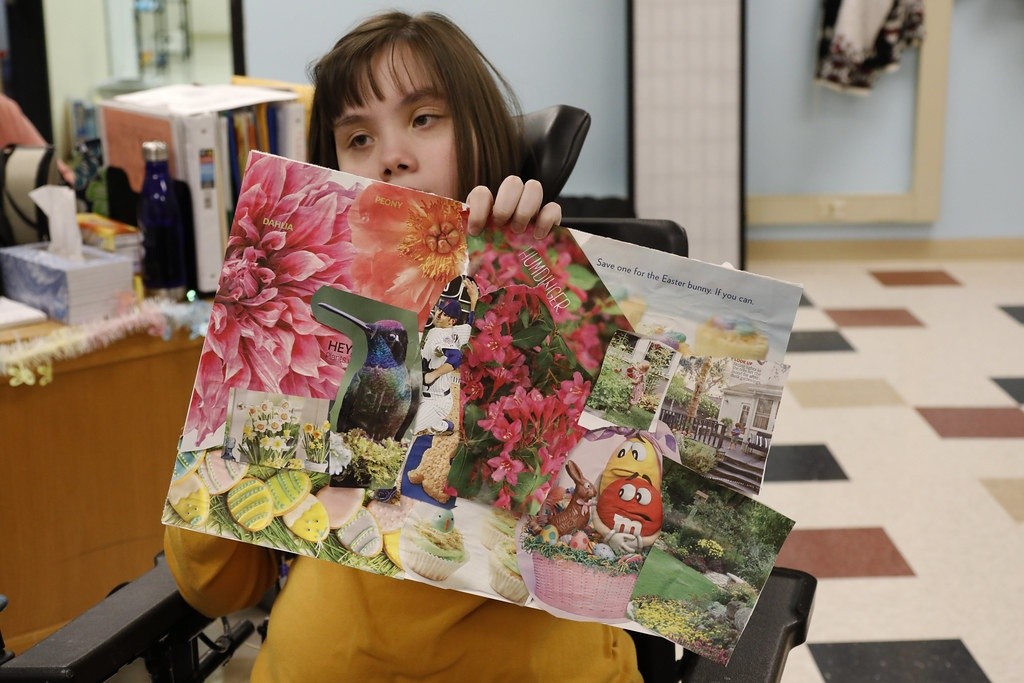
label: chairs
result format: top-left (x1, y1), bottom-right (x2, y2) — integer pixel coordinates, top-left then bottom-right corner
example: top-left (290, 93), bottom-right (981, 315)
top-left (0, 99), bottom-right (824, 683)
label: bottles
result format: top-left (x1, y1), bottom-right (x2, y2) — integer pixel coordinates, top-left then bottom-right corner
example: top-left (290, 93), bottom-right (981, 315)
top-left (137, 140), bottom-right (194, 303)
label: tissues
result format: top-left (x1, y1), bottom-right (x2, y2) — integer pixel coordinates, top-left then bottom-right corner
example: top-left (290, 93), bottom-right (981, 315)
top-left (0, 183), bottom-right (134, 326)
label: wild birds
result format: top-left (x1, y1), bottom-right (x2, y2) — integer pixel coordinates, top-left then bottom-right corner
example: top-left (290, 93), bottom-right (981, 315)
top-left (318, 303), bottom-right (420, 442)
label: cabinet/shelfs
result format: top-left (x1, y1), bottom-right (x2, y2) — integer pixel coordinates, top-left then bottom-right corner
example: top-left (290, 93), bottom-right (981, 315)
top-left (0, 293), bottom-right (221, 658)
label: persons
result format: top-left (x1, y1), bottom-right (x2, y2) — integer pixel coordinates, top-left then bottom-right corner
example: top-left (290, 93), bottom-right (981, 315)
top-left (410, 278), bottom-right (478, 435)
top-left (164, 11), bottom-right (644, 683)
